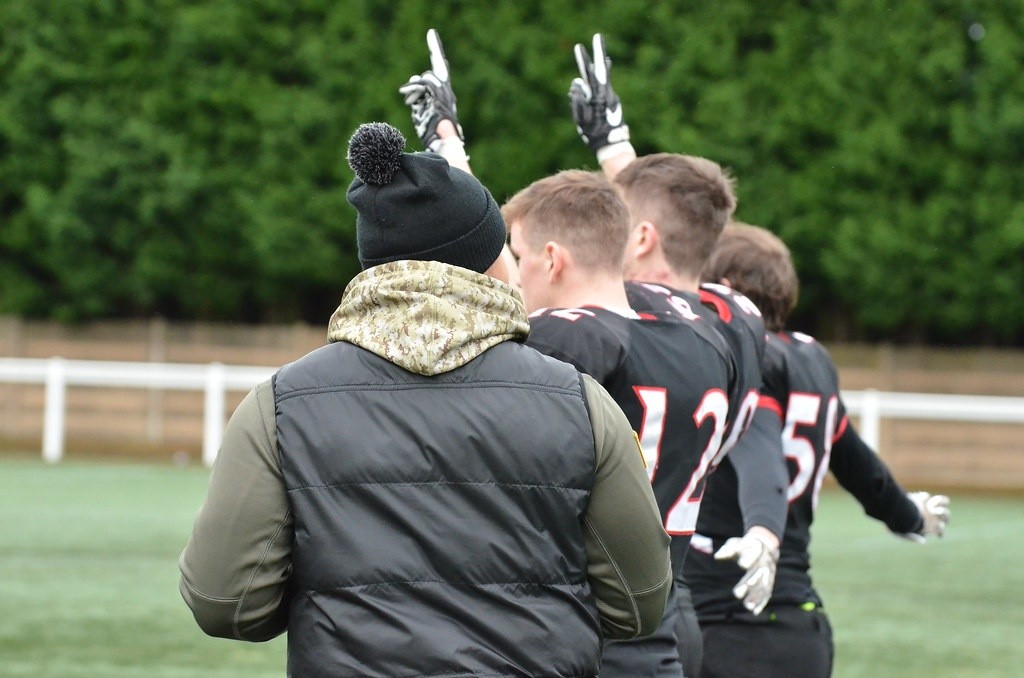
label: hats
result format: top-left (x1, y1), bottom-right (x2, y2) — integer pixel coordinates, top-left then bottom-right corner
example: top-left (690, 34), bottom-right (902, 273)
top-left (346, 122), bottom-right (506, 274)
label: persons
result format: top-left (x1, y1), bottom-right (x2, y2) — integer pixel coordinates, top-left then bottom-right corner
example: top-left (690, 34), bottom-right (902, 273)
top-left (179, 123), bottom-right (673, 676)
top-left (398, 27), bottom-right (950, 678)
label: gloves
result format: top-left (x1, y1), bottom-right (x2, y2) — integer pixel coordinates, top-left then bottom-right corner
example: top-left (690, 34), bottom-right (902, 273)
top-left (713, 526), bottom-right (780, 614)
top-left (898, 492), bottom-right (951, 546)
top-left (398, 29), bottom-right (465, 148)
top-left (566, 33), bottom-right (630, 155)
